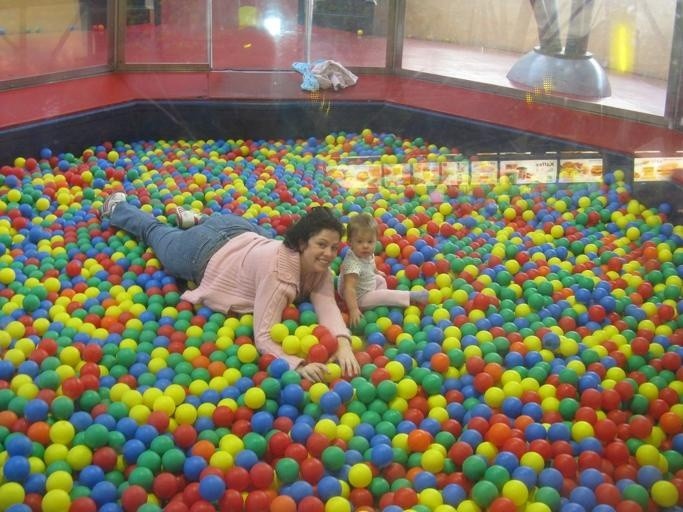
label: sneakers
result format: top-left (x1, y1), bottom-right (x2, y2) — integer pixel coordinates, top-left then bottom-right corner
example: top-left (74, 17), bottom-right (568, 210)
top-left (177, 205), bottom-right (199, 229)
top-left (101, 192), bottom-right (126, 219)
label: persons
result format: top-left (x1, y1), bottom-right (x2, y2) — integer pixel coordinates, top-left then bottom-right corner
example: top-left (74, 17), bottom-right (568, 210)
top-left (95, 190), bottom-right (360, 383)
top-left (338, 212), bottom-right (429, 329)
